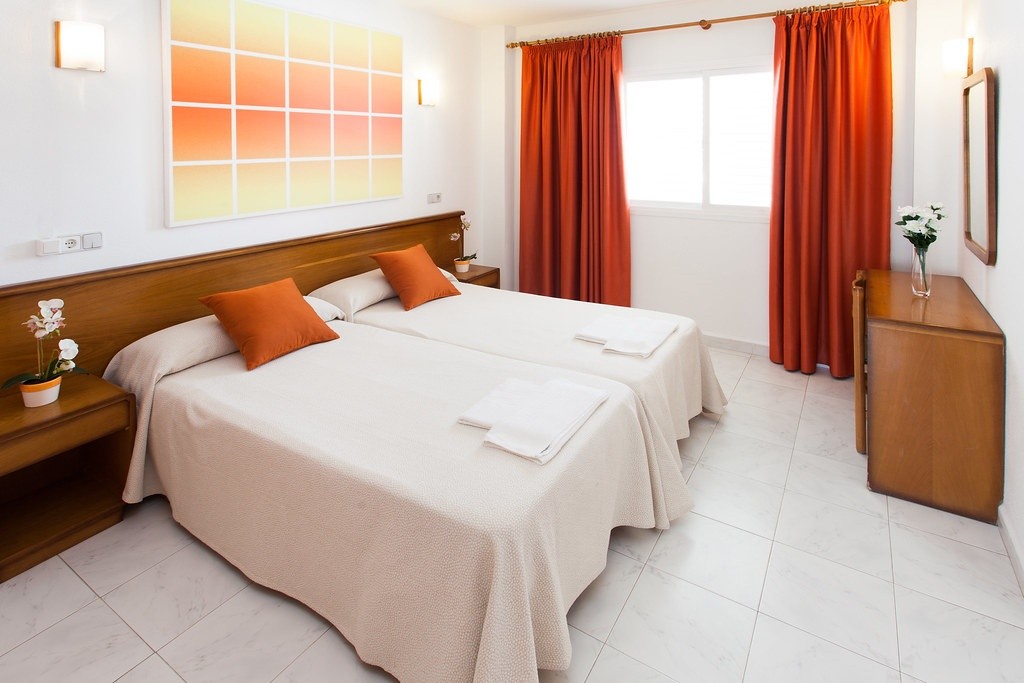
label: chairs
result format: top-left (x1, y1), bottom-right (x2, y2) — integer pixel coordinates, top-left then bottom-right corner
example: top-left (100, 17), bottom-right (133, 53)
top-left (852, 271), bottom-right (867, 455)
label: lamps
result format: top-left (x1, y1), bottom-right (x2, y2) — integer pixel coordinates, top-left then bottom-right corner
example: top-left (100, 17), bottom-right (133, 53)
top-left (56, 19), bottom-right (105, 73)
top-left (419, 80), bottom-right (437, 108)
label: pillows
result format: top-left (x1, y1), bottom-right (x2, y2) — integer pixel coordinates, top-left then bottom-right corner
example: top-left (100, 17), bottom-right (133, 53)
top-left (198, 278), bottom-right (340, 370)
top-left (368, 244), bottom-right (461, 311)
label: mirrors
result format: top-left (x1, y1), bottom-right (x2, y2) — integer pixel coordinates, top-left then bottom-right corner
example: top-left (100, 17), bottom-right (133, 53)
top-left (961, 67), bottom-right (997, 266)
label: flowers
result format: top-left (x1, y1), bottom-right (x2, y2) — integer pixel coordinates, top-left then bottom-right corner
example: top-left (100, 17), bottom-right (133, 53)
top-left (450, 215), bottom-right (478, 261)
top-left (2, 299), bottom-right (89, 390)
top-left (894, 203), bottom-right (948, 292)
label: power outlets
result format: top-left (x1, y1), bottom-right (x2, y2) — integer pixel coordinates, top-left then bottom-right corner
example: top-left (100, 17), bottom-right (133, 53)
top-left (59, 235), bottom-right (81, 252)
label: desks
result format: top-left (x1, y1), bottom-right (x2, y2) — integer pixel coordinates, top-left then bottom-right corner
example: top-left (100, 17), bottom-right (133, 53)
top-left (867, 267), bottom-right (1005, 526)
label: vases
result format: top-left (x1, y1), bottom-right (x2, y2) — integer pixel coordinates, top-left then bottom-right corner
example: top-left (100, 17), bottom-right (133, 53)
top-left (20, 373), bottom-right (62, 408)
top-left (911, 248), bottom-right (932, 297)
top-left (454, 258), bottom-right (472, 273)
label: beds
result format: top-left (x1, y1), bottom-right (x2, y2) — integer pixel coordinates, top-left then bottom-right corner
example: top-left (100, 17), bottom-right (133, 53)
top-left (102, 265), bottom-right (728, 683)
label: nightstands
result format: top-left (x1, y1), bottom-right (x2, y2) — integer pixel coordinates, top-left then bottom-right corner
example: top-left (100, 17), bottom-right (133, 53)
top-left (449, 264), bottom-right (500, 290)
top-left (0, 372), bottom-right (136, 584)
